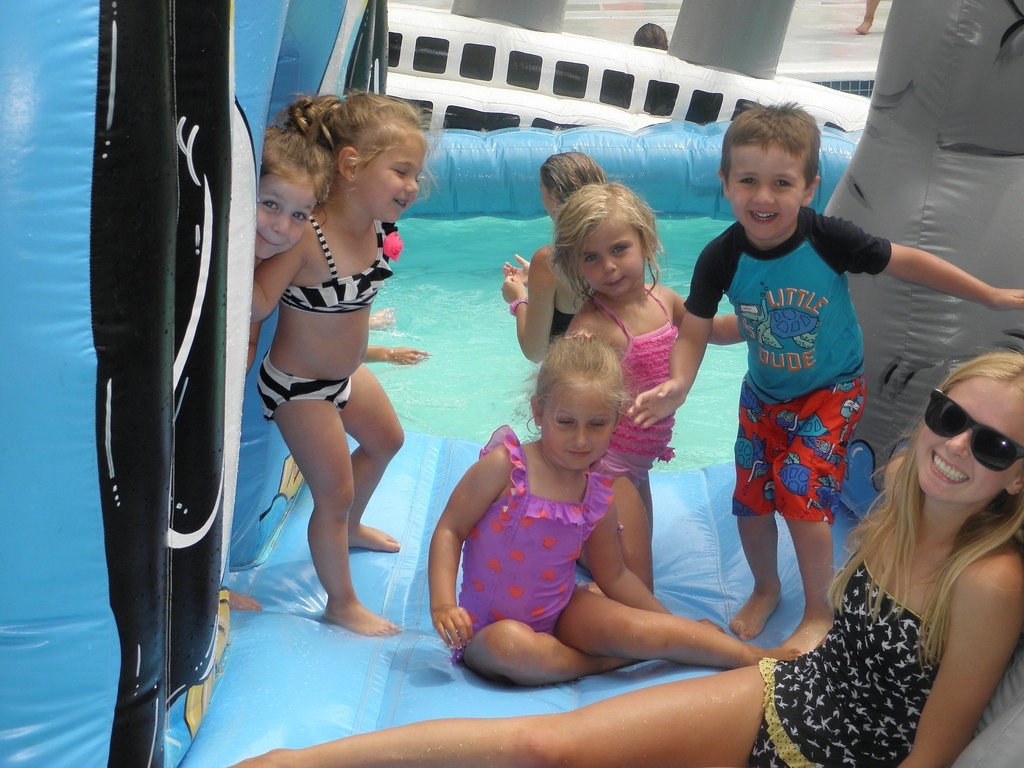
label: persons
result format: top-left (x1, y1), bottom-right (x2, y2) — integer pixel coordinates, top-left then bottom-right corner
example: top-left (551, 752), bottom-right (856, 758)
top-left (231, 350), bottom-right (1024, 768)
top-left (856, 0), bottom-right (880, 36)
top-left (360, 309), bottom-right (434, 364)
top-left (552, 185), bottom-right (747, 607)
top-left (503, 153), bottom-right (607, 361)
top-left (623, 102), bottom-right (1024, 642)
top-left (253, 90), bottom-right (429, 637)
top-left (429, 336), bottom-right (797, 686)
top-left (632, 23), bottom-right (668, 50)
top-left (225, 122), bottom-right (335, 613)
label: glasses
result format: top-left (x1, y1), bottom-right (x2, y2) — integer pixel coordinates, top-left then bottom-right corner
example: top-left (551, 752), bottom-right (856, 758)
top-left (925, 388), bottom-right (1024, 471)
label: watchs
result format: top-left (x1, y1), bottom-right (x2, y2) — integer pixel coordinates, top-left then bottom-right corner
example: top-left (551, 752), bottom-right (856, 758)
top-left (511, 298), bottom-right (528, 315)
